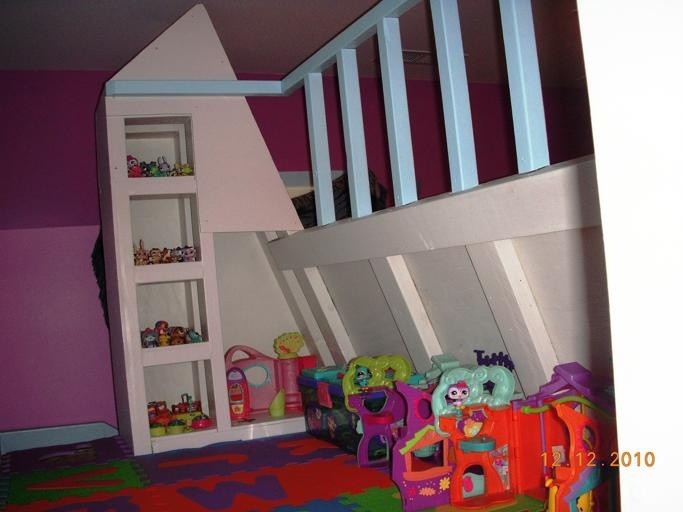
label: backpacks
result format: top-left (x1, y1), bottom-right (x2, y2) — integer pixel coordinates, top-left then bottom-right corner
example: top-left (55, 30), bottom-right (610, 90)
top-left (291, 168), bottom-right (387, 229)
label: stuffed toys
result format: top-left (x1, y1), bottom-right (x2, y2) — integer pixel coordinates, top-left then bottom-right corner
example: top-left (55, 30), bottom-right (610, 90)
top-left (138, 318), bottom-right (202, 350)
top-left (134, 238), bottom-right (197, 265)
top-left (127, 153), bottom-right (196, 177)
